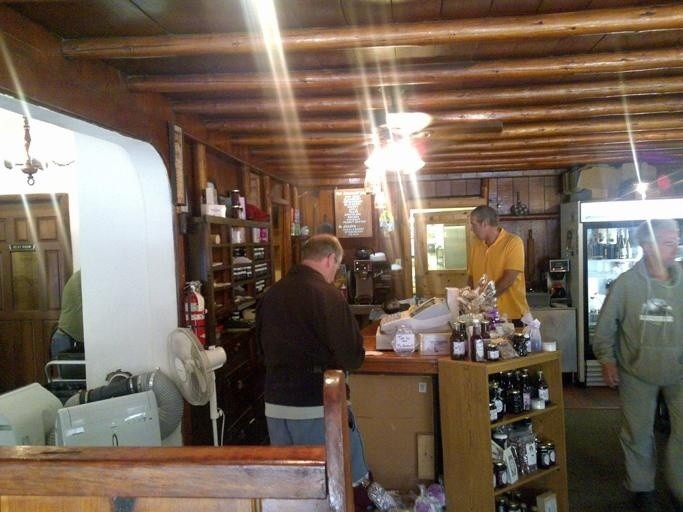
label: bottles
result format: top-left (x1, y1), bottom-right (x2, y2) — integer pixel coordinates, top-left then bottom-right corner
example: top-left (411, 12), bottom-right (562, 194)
top-left (590, 230), bottom-right (636, 261)
top-left (450, 315), bottom-right (559, 511)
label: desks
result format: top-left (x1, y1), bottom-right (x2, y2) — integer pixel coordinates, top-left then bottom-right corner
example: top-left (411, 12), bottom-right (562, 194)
top-left (342, 313), bottom-right (439, 495)
top-left (529, 302), bottom-right (577, 385)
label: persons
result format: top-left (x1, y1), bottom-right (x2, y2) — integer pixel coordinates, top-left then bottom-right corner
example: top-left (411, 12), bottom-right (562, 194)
top-left (49, 268), bottom-right (82, 363)
top-left (257, 235), bottom-right (370, 494)
top-left (592, 218), bottom-right (682, 510)
top-left (467, 204), bottom-right (531, 328)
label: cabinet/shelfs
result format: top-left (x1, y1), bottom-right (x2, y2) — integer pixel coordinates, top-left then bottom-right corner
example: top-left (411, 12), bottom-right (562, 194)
top-left (559, 199), bottom-right (682, 389)
top-left (437, 348), bottom-right (570, 511)
top-left (170, 127), bottom-right (401, 450)
top-left (480, 171), bottom-right (577, 294)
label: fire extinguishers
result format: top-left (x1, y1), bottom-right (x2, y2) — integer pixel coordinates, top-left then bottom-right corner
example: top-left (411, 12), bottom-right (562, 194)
top-left (183, 280), bottom-right (208, 347)
